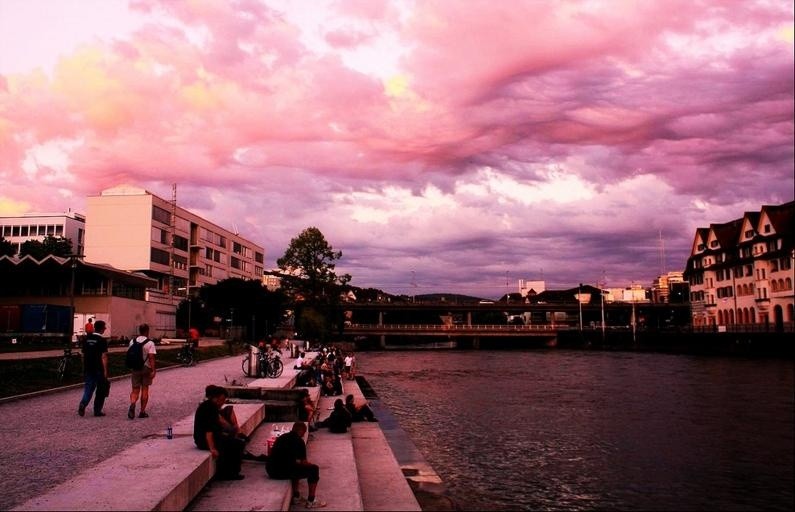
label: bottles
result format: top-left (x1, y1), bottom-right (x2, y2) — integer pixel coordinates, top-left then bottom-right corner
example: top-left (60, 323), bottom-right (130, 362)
top-left (168, 425), bottom-right (172, 438)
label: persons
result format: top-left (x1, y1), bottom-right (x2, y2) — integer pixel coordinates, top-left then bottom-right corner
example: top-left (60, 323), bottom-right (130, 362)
top-left (128, 324), bottom-right (156, 419)
top-left (79, 321), bottom-right (108, 416)
top-left (188, 326), bottom-right (200, 351)
top-left (194, 339), bottom-right (378, 508)
top-left (85, 318), bottom-right (94, 334)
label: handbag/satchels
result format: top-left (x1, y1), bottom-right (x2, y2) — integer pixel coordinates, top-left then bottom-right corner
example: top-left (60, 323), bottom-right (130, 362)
top-left (96, 378), bottom-right (111, 398)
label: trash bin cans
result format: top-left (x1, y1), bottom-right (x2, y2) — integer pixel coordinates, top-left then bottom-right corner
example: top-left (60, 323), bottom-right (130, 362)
top-left (247, 344), bottom-right (260, 377)
top-left (305, 340), bottom-right (309, 351)
top-left (290, 343), bottom-right (298, 357)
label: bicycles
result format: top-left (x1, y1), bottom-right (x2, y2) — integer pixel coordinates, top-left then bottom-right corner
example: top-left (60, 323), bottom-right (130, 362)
top-left (177, 344), bottom-right (193, 365)
top-left (241, 351), bottom-right (283, 378)
top-left (58, 341), bottom-right (80, 380)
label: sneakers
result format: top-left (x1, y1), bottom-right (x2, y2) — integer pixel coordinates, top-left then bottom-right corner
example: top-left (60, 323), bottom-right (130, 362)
top-left (128, 404), bottom-right (136, 420)
top-left (94, 412), bottom-right (106, 416)
top-left (305, 497), bottom-right (327, 508)
top-left (292, 495), bottom-right (308, 505)
top-left (78, 402), bottom-right (85, 416)
top-left (138, 411), bottom-right (148, 418)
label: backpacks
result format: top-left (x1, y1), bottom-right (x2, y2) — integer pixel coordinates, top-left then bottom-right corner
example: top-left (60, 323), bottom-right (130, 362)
top-left (123, 338), bottom-right (151, 372)
